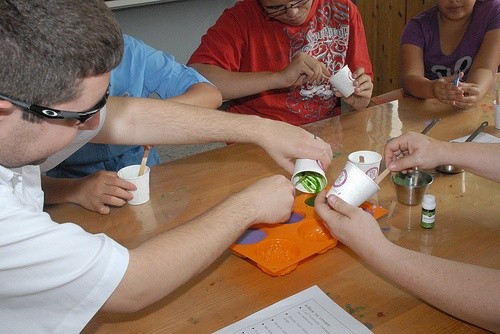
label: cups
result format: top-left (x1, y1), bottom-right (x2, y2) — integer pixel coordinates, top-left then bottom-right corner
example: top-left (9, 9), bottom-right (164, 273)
top-left (328, 65), bottom-right (356, 98)
top-left (435, 164), bottom-right (465, 174)
top-left (291, 159), bottom-right (327, 193)
top-left (326, 161), bottom-right (381, 207)
top-left (392, 170), bottom-right (434, 205)
top-left (117, 164), bottom-right (150, 206)
top-left (348, 150), bottom-right (382, 180)
top-left (492, 99), bottom-right (500, 129)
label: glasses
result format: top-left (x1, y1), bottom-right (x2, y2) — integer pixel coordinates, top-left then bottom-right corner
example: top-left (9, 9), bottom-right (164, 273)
top-left (260, 0), bottom-right (309, 18)
top-left (0, 84), bottom-right (112, 126)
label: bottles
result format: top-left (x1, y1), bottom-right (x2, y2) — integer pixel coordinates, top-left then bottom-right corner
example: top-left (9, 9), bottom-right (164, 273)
top-left (421, 195), bottom-right (436, 228)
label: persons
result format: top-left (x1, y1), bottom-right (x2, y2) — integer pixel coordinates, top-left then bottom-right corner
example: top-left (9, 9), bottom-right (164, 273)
top-left (0, 0), bottom-right (335, 334)
top-left (185, 0), bottom-right (375, 128)
top-left (313, 131), bottom-right (500, 334)
top-left (365, 0), bottom-right (500, 111)
top-left (38, 33), bottom-right (224, 215)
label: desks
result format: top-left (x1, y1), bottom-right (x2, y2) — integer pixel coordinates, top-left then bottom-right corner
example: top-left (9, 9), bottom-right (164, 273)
top-left (43, 72), bottom-right (499, 334)
